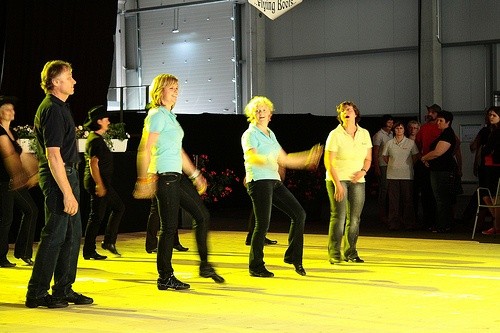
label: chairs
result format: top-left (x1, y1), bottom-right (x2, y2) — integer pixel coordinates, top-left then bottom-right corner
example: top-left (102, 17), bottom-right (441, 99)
top-left (470, 178), bottom-right (500, 239)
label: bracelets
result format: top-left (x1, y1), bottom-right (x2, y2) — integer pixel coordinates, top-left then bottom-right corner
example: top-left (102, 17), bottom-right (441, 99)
top-left (188, 168), bottom-right (201, 180)
top-left (136, 177), bottom-right (151, 184)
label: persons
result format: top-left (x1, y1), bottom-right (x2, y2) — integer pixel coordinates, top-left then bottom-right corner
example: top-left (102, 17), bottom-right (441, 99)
top-left (372, 114), bottom-right (394, 227)
top-left (469, 106), bottom-right (500, 236)
top-left (23, 60), bottom-right (95, 309)
top-left (1, 97), bottom-right (35, 267)
top-left (414, 104), bottom-right (464, 233)
top-left (420, 109), bottom-right (456, 234)
top-left (241, 96), bottom-right (324, 278)
top-left (323, 101), bottom-right (373, 264)
top-left (81, 105), bottom-right (122, 261)
top-left (405, 120), bottom-right (430, 230)
top-left (131, 73), bottom-right (226, 290)
top-left (145, 196), bottom-right (190, 254)
top-left (383, 122), bottom-right (415, 235)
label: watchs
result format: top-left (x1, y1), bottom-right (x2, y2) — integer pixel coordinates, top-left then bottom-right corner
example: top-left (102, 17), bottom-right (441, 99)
top-left (361, 169), bottom-right (368, 173)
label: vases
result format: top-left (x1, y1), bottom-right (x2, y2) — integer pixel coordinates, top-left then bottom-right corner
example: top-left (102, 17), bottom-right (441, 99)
top-left (16, 140), bottom-right (129, 153)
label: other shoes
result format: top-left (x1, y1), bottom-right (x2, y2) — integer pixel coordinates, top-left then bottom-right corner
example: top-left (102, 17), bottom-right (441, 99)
top-left (431, 227), bottom-right (500, 236)
top-left (329, 256), bottom-right (364, 264)
top-left (0, 255), bottom-right (16, 267)
top-left (147, 243), bottom-right (189, 253)
top-left (101, 240), bottom-right (121, 256)
top-left (84, 251), bottom-right (107, 259)
top-left (16, 253), bottom-right (34, 266)
top-left (246, 235), bottom-right (277, 246)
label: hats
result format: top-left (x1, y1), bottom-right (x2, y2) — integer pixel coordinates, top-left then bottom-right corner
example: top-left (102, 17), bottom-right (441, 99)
top-left (88, 105), bottom-right (106, 120)
top-left (426, 104), bottom-right (441, 113)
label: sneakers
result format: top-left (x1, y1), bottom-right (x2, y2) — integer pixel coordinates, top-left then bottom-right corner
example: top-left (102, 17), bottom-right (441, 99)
top-left (25, 293), bottom-right (69, 308)
top-left (157, 274), bottom-right (190, 290)
top-left (283, 258), bottom-right (306, 276)
top-left (52, 288), bottom-right (93, 304)
top-left (248, 266), bottom-right (274, 277)
top-left (200, 266), bottom-right (224, 283)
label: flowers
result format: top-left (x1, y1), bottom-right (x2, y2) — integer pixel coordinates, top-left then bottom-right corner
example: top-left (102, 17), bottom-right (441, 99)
top-left (10, 124), bottom-right (131, 139)
